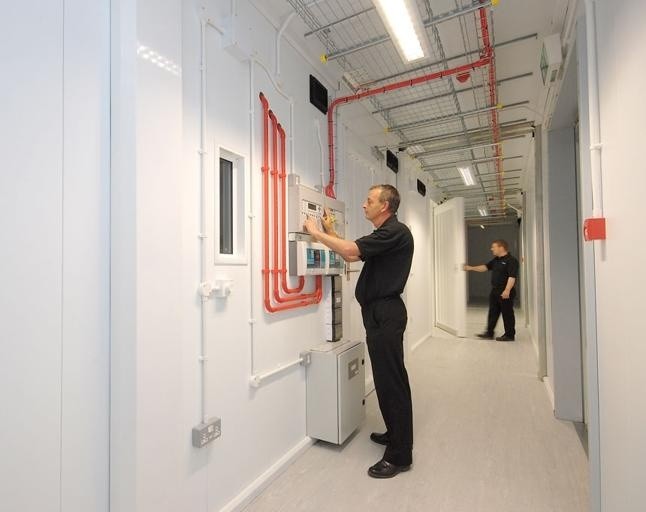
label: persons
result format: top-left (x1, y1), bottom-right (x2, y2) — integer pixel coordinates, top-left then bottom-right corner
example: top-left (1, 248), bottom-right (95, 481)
top-left (304, 183), bottom-right (414, 478)
top-left (463, 239), bottom-right (519, 342)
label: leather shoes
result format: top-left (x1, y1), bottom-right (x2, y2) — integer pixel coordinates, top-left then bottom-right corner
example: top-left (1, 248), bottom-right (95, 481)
top-left (367, 458), bottom-right (410, 479)
top-left (370, 432), bottom-right (386, 445)
top-left (495, 333), bottom-right (515, 341)
top-left (478, 332), bottom-right (494, 339)
